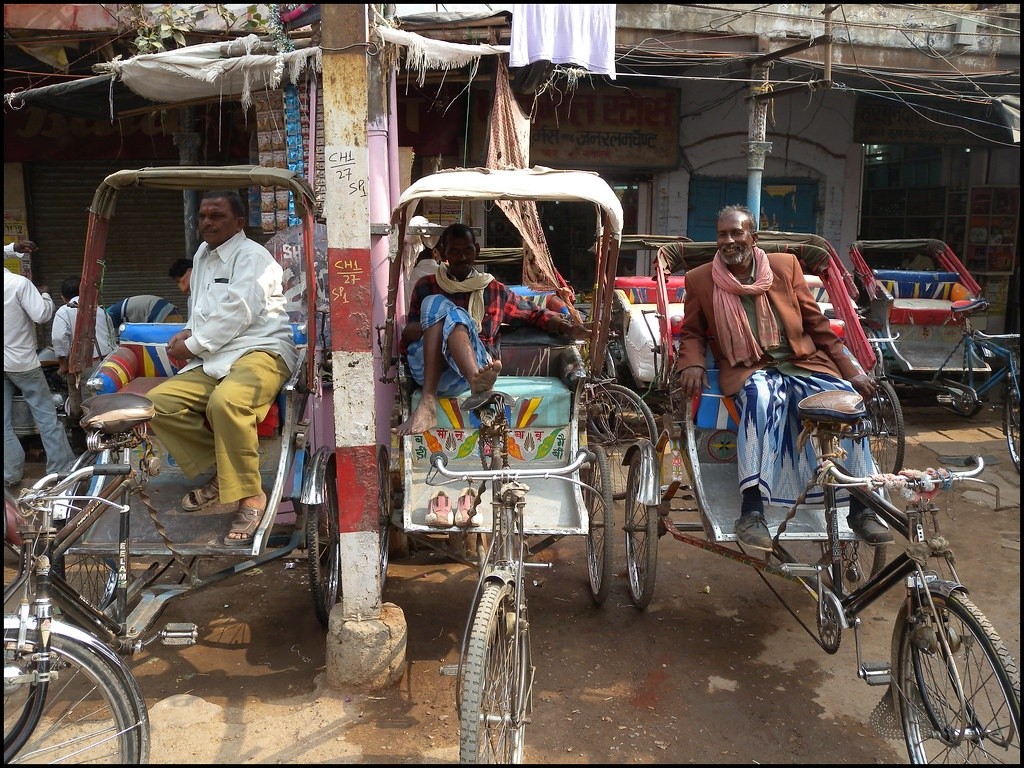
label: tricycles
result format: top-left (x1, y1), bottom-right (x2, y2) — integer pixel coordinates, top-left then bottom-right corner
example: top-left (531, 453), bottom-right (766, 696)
top-left (474, 230), bottom-right (1023, 501)
top-left (376, 168), bottom-right (614, 764)
top-left (5, 164), bottom-right (336, 763)
top-left (621, 243), bottom-right (1021, 764)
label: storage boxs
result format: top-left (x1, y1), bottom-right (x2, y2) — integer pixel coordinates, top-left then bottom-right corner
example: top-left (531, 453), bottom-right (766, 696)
top-left (903, 145), bottom-right (1021, 188)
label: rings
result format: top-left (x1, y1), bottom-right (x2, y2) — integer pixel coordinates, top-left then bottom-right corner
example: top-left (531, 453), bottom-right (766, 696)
top-left (870, 380), bottom-right (873, 384)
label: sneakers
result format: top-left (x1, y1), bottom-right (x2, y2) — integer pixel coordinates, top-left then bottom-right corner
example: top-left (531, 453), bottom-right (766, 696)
top-left (847, 510), bottom-right (895, 547)
top-left (734, 515), bottom-right (774, 553)
top-left (425, 490), bottom-right (453, 528)
top-left (455, 488), bottom-right (482, 527)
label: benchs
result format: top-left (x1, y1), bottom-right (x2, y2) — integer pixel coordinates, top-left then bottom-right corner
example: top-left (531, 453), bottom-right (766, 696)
top-left (406, 345), bottom-right (586, 428)
top-left (689, 319), bottom-right (848, 433)
top-left (872, 269), bottom-right (976, 324)
top-left (86, 321), bottom-right (308, 438)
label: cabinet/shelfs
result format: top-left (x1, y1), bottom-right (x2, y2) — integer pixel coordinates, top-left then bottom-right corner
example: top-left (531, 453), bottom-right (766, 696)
top-left (858, 144), bottom-right (1021, 276)
top-left (485, 187), bottom-right (639, 292)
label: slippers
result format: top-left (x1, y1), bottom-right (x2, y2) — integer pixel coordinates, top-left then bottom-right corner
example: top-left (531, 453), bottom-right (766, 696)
top-left (179, 480), bottom-right (222, 512)
top-left (223, 505), bottom-right (265, 546)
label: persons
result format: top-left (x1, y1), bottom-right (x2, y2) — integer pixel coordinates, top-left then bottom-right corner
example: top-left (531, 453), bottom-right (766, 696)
top-left (4, 240), bottom-right (193, 488)
top-left (405, 245), bottom-right (444, 316)
top-left (144, 189), bottom-right (295, 545)
top-left (676, 205), bottom-right (895, 551)
top-left (391, 224), bottom-right (602, 436)
